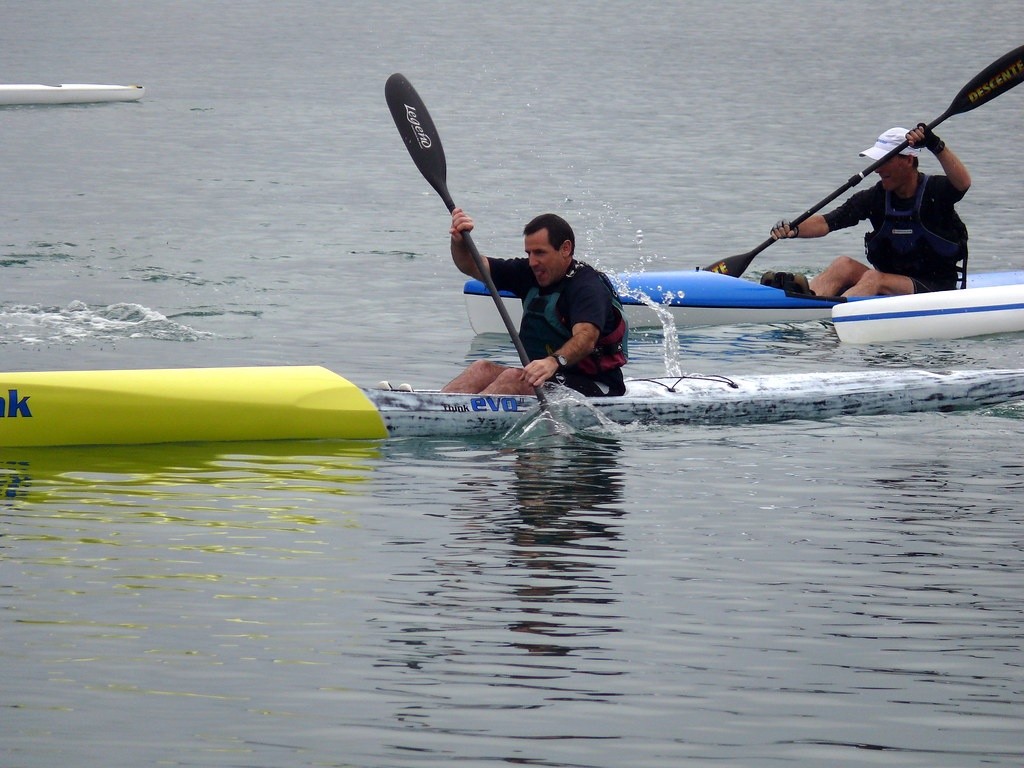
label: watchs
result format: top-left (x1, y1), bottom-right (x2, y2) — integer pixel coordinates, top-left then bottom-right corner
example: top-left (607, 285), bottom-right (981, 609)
top-left (551, 354), bottom-right (568, 369)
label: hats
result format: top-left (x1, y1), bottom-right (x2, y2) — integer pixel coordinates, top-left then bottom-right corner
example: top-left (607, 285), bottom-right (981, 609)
top-left (859, 127), bottom-right (922, 161)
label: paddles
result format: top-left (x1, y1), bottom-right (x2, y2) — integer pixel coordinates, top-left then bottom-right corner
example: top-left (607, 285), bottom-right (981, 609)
top-left (699, 44), bottom-right (1024, 279)
top-left (383, 72), bottom-right (553, 419)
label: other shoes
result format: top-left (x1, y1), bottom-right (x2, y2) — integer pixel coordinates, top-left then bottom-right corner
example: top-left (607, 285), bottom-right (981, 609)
top-left (377, 381), bottom-right (391, 391)
top-left (397, 383), bottom-right (412, 393)
top-left (793, 271), bottom-right (814, 297)
top-left (760, 270), bottom-right (775, 288)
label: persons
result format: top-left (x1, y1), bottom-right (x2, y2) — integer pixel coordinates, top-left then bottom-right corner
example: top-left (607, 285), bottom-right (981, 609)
top-left (442, 206), bottom-right (628, 396)
top-left (760, 124), bottom-right (971, 298)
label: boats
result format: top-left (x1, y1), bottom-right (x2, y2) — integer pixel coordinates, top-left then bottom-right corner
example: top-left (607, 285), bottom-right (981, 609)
top-left (831, 284), bottom-right (1024, 343)
top-left (0, 83), bottom-right (147, 106)
top-left (0, 364), bottom-right (1024, 448)
top-left (463, 271), bottom-right (1024, 337)
